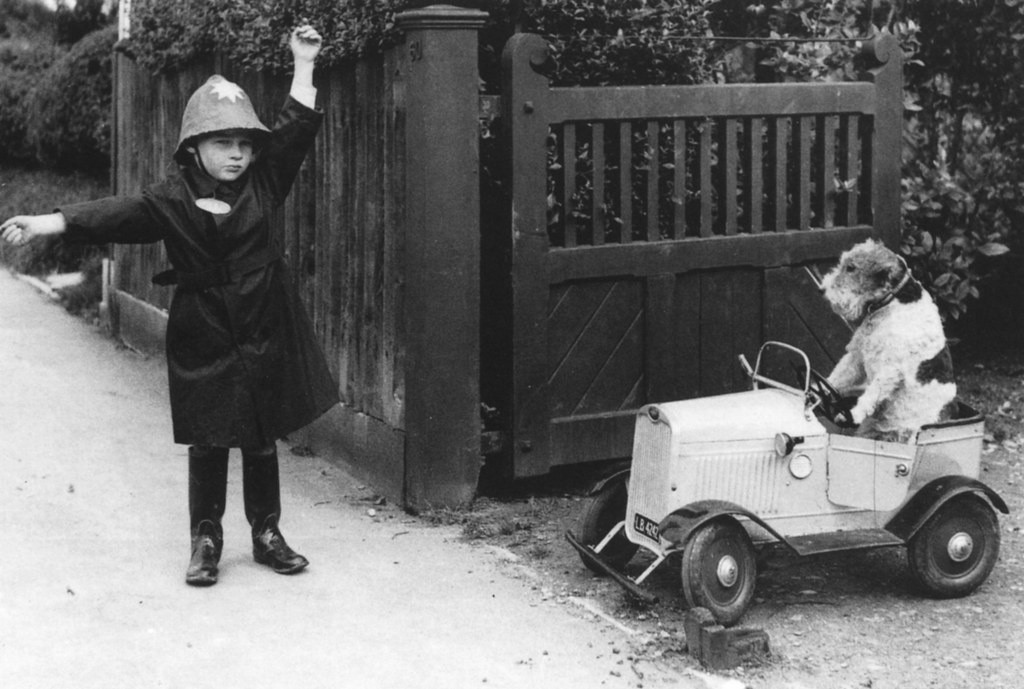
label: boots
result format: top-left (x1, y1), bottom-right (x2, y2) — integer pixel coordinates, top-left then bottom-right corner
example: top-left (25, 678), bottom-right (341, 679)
top-left (242, 451), bottom-right (309, 573)
top-left (185, 445), bottom-right (228, 585)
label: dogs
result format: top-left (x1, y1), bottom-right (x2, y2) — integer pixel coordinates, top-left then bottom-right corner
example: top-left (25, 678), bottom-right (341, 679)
top-left (818, 237), bottom-right (961, 445)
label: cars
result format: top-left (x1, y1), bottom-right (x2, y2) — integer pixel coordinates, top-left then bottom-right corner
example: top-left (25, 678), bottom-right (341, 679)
top-left (564, 340), bottom-right (1008, 626)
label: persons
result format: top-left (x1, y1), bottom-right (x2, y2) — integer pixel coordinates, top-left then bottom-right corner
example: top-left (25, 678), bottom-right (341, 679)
top-left (0, 19), bottom-right (339, 585)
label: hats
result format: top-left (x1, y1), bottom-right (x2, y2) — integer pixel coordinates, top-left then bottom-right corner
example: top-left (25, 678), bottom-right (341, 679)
top-left (173, 74), bottom-right (272, 167)
